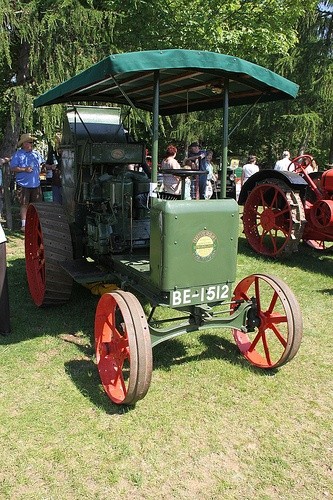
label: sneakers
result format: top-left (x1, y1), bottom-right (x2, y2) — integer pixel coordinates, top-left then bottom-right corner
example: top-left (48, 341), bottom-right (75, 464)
top-left (20, 226), bottom-right (26, 232)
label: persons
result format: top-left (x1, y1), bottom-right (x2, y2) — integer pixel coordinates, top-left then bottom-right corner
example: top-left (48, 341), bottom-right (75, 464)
top-left (10, 134), bottom-right (46, 233)
top-left (273, 151), bottom-right (295, 172)
top-left (302, 159), bottom-right (318, 177)
top-left (241, 156), bottom-right (259, 186)
top-left (45, 133), bottom-right (63, 202)
top-left (128, 145), bottom-right (218, 200)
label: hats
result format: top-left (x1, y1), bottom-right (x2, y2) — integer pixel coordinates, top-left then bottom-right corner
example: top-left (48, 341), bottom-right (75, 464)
top-left (15, 134), bottom-right (37, 149)
top-left (189, 142), bottom-right (198, 146)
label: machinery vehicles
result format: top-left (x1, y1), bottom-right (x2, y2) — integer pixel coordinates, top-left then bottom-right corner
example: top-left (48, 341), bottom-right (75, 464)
top-left (23, 47), bottom-right (304, 406)
top-left (238, 155), bottom-right (333, 259)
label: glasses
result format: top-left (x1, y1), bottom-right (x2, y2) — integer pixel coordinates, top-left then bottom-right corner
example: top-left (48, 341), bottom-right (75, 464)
top-left (191, 147), bottom-right (200, 149)
top-left (27, 141), bottom-right (33, 143)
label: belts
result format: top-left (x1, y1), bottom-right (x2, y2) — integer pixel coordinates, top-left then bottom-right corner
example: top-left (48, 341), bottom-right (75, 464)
top-left (22, 185), bottom-right (39, 189)
